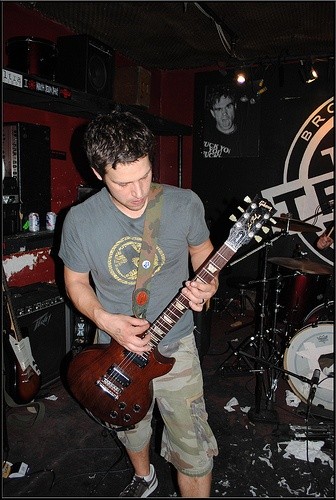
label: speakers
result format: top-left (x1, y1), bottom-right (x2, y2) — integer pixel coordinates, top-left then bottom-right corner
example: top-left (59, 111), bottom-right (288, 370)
top-left (57, 34), bottom-right (114, 100)
top-left (3, 281), bottom-right (72, 391)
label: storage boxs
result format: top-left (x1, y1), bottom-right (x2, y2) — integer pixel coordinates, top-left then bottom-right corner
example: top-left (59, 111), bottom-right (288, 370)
top-left (113, 66), bottom-right (153, 110)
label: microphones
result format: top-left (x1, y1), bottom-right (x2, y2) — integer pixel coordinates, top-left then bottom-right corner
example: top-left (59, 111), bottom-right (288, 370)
top-left (309, 369), bottom-right (320, 401)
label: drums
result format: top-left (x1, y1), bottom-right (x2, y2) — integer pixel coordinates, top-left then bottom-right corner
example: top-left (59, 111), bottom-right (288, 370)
top-left (264, 257), bottom-right (330, 311)
top-left (284, 300), bottom-right (336, 424)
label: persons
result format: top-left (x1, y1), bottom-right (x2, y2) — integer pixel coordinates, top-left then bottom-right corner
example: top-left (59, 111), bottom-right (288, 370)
top-left (208, 85), bottom-right (243, 147)
top-left (58, 111), bottom-right (219, 498)
top-left (317, 224), bottom-right (335, 251)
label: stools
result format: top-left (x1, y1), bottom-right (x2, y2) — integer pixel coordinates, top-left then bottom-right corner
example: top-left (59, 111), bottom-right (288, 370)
top-left (221, 277), bottom-right (257, 317)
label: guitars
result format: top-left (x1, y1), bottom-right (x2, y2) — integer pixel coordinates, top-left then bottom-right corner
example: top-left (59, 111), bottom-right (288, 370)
top-left (67, 191), bottom-right (284, 433)
top-left (2, 256), bottom-right (45, 408)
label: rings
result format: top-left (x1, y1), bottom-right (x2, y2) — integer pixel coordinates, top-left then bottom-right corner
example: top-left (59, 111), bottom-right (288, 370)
top-left (199, 299), bottom-right (205, 304)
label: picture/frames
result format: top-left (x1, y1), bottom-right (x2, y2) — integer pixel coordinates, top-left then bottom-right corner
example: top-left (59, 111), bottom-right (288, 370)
top-left (201, 83), bottom-right (261, 158)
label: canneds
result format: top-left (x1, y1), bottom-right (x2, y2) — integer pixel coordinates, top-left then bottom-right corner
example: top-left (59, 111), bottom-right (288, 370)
top-left (46, 211), bottom-right (56, 230)
top-left (28, 212), bottom-right (39, 232)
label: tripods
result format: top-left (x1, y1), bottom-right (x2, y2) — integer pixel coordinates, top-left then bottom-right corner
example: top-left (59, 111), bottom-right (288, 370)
top-left (210, 206), bottom-right (335, 413)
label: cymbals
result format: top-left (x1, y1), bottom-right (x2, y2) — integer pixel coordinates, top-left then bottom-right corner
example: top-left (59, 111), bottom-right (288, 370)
top-left (264, 216), bottom-right (323, 233)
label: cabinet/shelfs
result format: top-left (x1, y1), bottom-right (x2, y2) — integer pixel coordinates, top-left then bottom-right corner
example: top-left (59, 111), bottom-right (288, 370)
top-left (3, 69), bottom-right (193, 254)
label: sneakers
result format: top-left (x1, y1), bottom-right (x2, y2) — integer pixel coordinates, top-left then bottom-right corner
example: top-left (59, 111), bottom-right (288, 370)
top-left (122, 464), bottom-right (159, 496)
